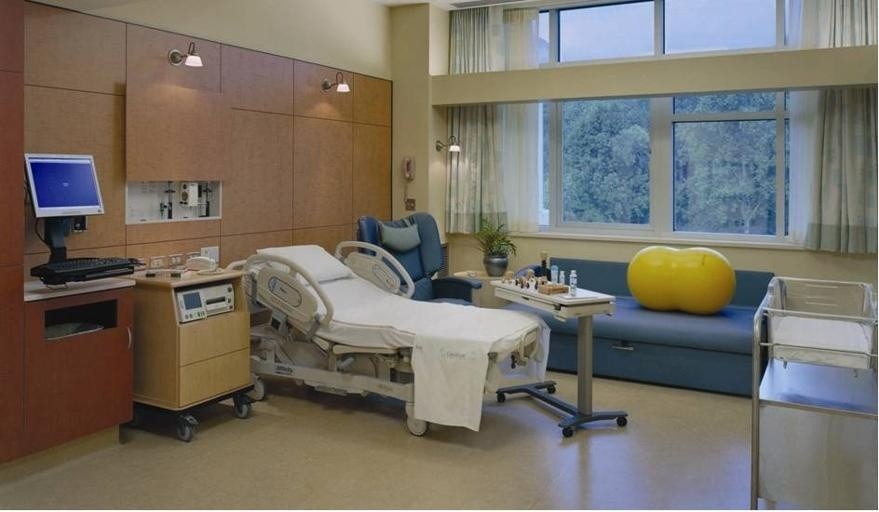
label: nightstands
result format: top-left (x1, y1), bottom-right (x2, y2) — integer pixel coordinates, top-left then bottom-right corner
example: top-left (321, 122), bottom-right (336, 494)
top-left (122, 265), bottom-right (254, 440)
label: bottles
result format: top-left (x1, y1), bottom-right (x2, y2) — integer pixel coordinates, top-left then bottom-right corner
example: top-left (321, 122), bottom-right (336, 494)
top-left (551, 266), bottom-right (558, 283)
top-left (530, 276), bottom-right (535, 288)
top-left (559, 271), bottom-right (565, 285)
top-left (569, 270), bottom-right (577, 298)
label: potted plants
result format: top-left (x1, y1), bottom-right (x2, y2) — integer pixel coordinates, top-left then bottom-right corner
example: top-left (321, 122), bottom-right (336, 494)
top-left (470, 213), bottom-right (517, 277)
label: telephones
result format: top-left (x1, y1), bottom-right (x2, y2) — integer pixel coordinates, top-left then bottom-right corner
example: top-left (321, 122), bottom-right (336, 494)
top-left (185, 257), bottom-right (218, 272)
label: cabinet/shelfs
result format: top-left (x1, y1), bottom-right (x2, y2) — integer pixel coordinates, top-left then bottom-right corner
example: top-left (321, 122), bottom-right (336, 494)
top-left (24, 285), bottom-right (137, 456)
top-left (453, 268), bottom-right (513, 310)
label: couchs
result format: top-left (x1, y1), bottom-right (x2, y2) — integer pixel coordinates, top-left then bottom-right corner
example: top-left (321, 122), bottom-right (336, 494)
top-left (505, 256), bottom-right (775, 397)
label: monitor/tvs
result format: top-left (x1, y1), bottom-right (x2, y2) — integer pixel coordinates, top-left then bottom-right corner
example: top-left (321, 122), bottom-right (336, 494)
top-left (21, 153), bottom-right (105, 261)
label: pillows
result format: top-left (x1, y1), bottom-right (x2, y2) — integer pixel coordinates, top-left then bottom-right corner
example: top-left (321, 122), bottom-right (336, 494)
top-left (256, 242), bottom-right (354, 285)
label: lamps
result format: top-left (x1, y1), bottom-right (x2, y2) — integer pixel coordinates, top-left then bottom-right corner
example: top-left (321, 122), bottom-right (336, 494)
top-left (436, 134), bottom-right (462, 153)
top-left (320, 70), bottom-right (350, 93)
top-left (168, 40), bottom-right (203, 69)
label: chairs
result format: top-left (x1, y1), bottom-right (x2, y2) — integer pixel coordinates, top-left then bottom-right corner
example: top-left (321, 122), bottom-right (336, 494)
top-left (356, 211), bottom-right (483, 306)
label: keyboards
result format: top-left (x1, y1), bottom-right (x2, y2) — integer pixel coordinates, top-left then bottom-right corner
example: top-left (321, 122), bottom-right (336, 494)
top-left (31, 256), bottom-right (135, 284)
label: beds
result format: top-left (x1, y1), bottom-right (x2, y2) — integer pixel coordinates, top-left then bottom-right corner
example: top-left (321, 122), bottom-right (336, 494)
top-left (226, 240), bottom-right (550, 438)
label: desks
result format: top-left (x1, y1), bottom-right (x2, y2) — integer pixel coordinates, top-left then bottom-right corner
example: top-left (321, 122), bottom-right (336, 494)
top-left (489, 278), bottom-right (627, 437)
